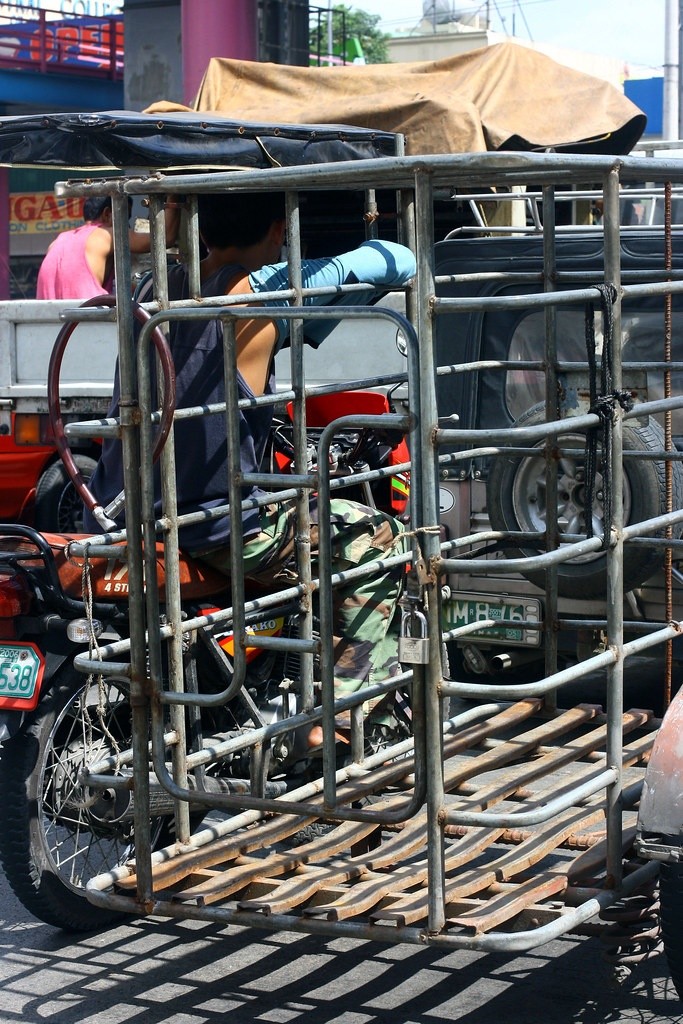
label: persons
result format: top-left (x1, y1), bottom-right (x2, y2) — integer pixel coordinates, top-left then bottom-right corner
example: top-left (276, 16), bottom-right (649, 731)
top-left (35, 188), bottom-right (182, 300)
top-left (79, 193), bottom-right (418, 747)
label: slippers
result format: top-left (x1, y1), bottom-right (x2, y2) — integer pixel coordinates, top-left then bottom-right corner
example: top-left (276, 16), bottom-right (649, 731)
top-left (304, 718), bottom-right (392, 771)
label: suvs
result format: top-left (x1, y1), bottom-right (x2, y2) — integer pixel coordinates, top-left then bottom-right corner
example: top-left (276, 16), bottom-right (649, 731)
top-left (422, 225), bottom-right (683, 708)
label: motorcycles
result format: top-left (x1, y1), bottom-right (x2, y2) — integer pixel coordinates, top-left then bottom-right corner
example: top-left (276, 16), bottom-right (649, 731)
top-left (0, 321), bottom-right (450, 928)
